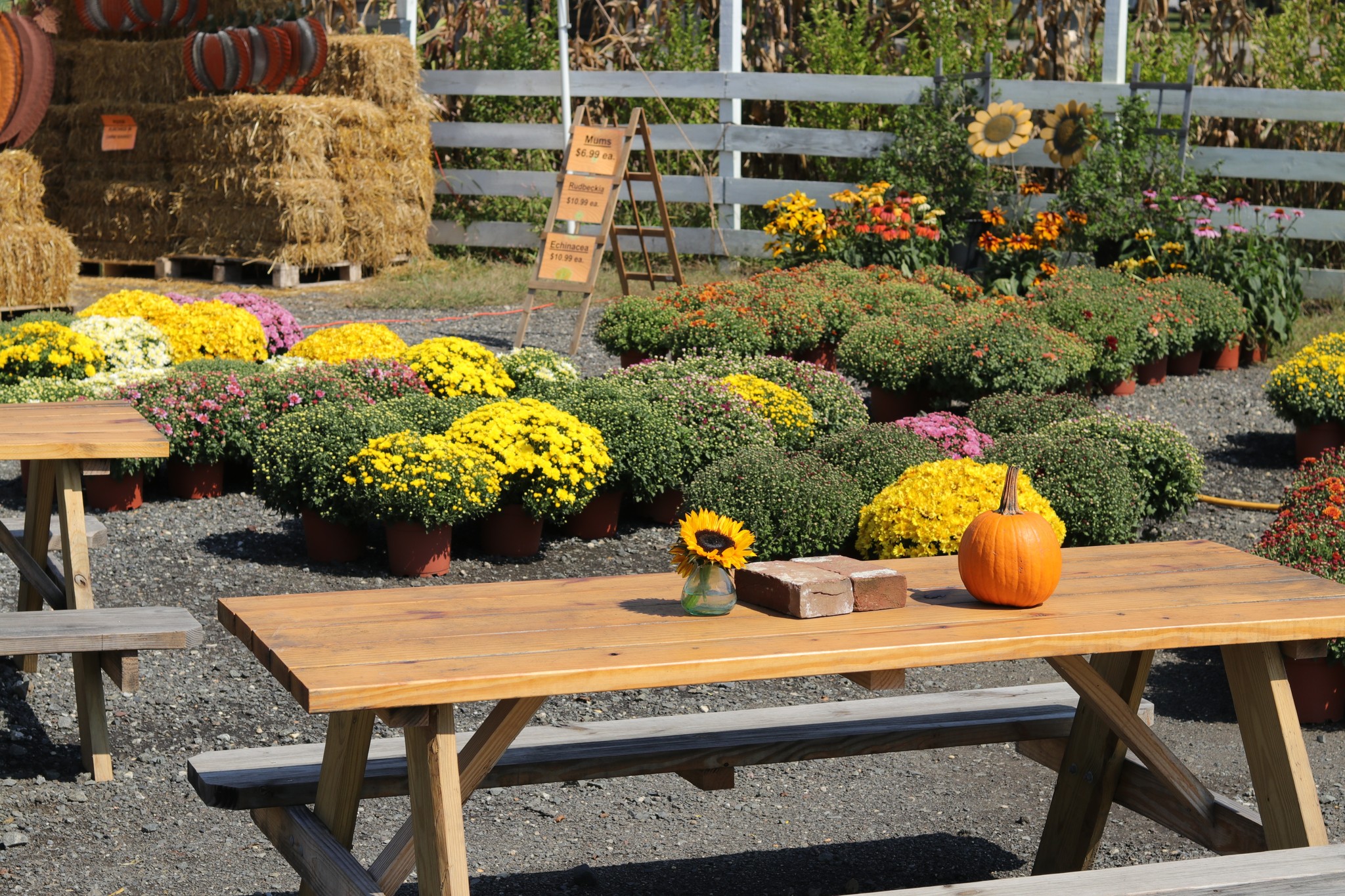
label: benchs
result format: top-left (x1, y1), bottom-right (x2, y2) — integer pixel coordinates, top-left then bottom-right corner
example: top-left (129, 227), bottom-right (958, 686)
top-left (184, 677), bottom-right (1156, 814)
top-left (0, 603), bottom-right (204, 658)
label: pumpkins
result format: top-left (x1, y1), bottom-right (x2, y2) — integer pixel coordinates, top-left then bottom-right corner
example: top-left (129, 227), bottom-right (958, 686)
top-left (958, 464), bottom-right (1062, 608)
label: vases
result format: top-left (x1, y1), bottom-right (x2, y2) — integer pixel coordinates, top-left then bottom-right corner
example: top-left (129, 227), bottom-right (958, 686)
top-left (1283, 650), bottom-right (1345, 729)
top-left (173, 456), bottom-right (225, 501)
top-left (1101, 368), bottom-right (1139, 397)
top-left (1170, 346), bottom-right (1203, 378)
top-left (1291, 410), bottom-right (1343, 469)
top-left (1246, 333), bottom-right (1269, 367)
top-left (1205, 327), bottom-right (1245, 373)
top-left (618, 342), bottom-right (653, 372)
top-left (678, 560), bottom-right (738, 618)
top-left (478, 501), bottom-right (544, 559)
top-left (82, 463), bottom-right (147, 516)
top-left (297, 503), bottom-right (364, 567)
top-left (640, 483), bottom-right (687, 529)
top-left (796, 338), bottom-right (839, 375)
top-left (15, 454), bottom-right (57, 504)
top-left (863, 380), bottom-right (919, 427)
top-left (564, 487), bottom-right (624, 542)
top-left (1134, 355), bottom-right (1169, 387)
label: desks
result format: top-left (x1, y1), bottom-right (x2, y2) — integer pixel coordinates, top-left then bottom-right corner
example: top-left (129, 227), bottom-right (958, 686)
top-left (0, 398), bottom-right (171, 785)
top-left (213, 538), bottom-right (1345, 896)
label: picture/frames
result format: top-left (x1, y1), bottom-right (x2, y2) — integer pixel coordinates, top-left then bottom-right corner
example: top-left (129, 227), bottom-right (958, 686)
top-left (382, 516), bottom-right (455, 580)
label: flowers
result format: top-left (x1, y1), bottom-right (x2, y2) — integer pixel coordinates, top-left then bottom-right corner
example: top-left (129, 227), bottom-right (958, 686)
top-left (966, 97), bottom-right (1035, 237)
top-left (0, 177), bottom-right (1345, 614)
top-left (1037, 97), bottom-right (1100, 172)
top-left (1324, 636), bottom-right (1345, 659)
